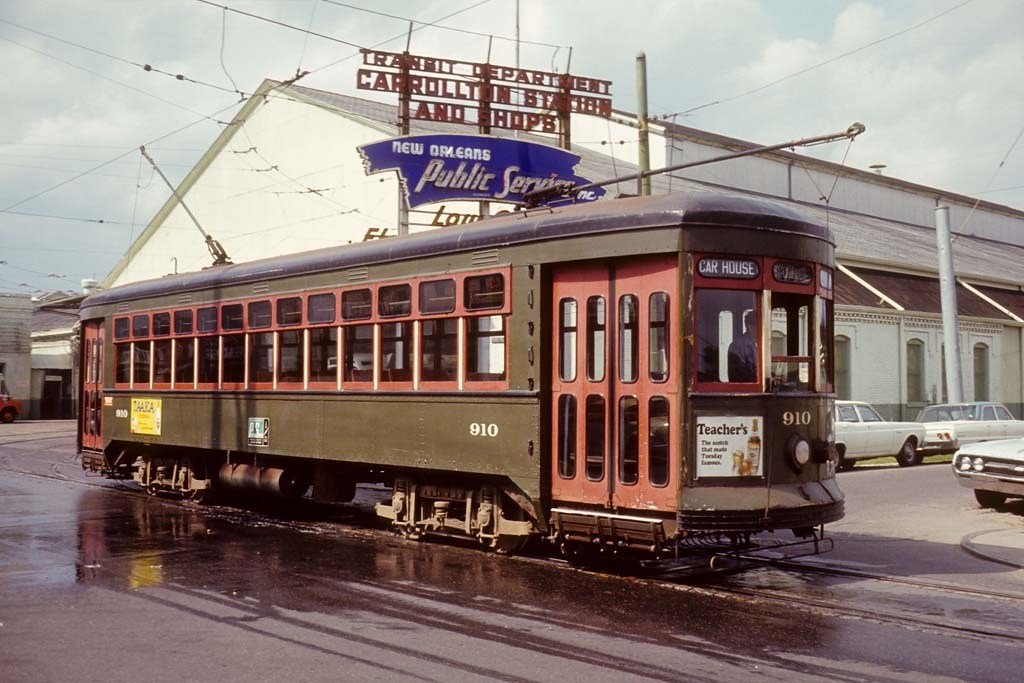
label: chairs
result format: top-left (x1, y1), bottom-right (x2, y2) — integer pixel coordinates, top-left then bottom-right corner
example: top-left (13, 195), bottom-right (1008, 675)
top-left (256, 366), bottom-right (502, 382)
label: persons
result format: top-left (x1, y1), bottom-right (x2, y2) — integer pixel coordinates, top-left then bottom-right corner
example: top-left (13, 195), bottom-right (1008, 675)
top-left (727, 310), bottom-right (757, 381)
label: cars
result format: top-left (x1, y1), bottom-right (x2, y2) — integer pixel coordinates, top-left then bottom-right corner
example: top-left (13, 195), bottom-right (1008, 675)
top-left (951, 438), bottom-right (1024, 508)
top-left (913, 401), bottom-right (1024, 456)
top-left (834, 399), bottom-right (927, 475)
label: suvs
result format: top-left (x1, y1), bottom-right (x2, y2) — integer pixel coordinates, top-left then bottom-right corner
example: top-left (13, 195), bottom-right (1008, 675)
top-left (0, 373), bottom-right (23, 424)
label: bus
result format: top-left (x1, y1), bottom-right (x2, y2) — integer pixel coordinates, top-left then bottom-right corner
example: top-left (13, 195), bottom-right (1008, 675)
top-left (75, 185), bottom-right (849, 578)
